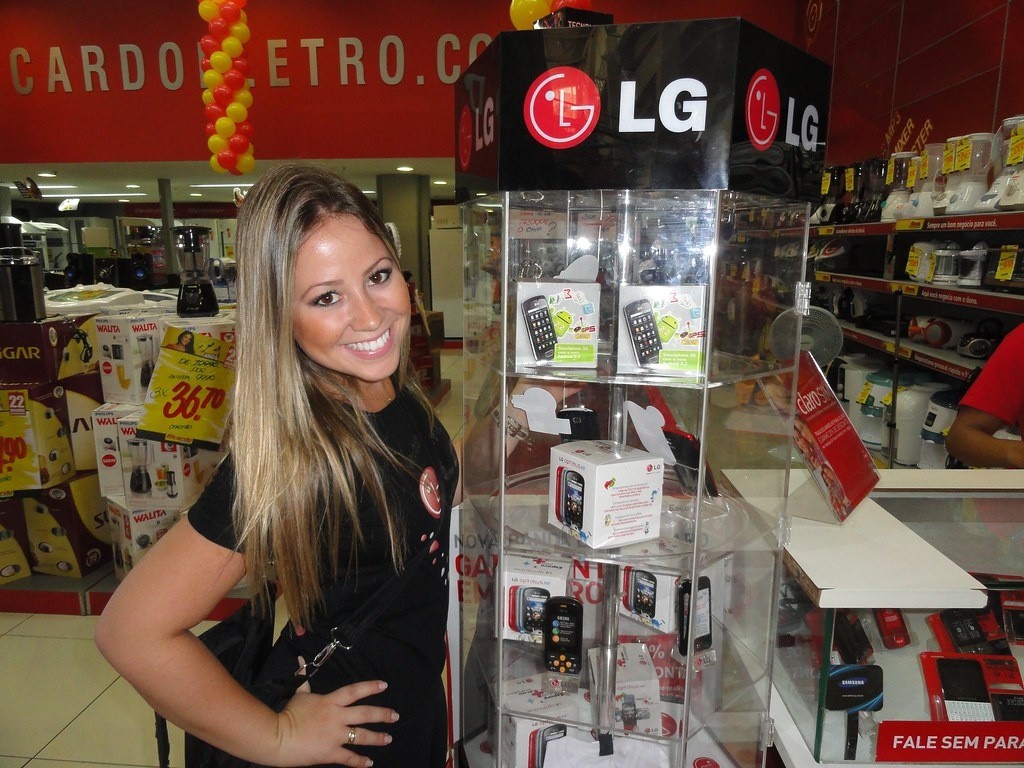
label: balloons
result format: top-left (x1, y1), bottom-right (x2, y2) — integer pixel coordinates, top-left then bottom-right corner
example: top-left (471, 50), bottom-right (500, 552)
top-left (509, 0), bottom-right (591, 32)
top-left (198, 0), bottom-right (255, 177)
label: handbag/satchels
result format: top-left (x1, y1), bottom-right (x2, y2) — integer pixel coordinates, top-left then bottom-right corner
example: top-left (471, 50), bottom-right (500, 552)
top-left (184, 582), bottom-right (328, 767)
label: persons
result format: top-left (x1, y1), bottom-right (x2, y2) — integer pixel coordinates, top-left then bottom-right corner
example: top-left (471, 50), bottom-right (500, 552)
top-left (761, 376), bottom-right (852, 522)
top-left (944, 321), bottom-right (1024, 468)
top-left (166, 331), bottom-right (194, 354)
top-left (92, 163), bottom-right (587, 768)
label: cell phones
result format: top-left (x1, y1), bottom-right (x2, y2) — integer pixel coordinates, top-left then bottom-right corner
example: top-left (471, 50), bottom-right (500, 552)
top-left (522, 295), bottom-right (558, 361)
top-left (624, 298), bottom-right (663, 367)
top-left (554, 466), bottom-right (585, 531)
top-left (816, 606), bottom-right (1023, 723)
top-left (675, 573), bottom-right (713, 656)
top-left (626, 568), bottom-right (657, 622)
top-left (508, 587), bottom-right (549, 638)
top-left (542, 596), bottom-right (586, 677)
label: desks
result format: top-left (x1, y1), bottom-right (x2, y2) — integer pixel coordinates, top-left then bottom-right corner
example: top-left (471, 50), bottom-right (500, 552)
top-left (720, 467), bottom-right (1023, 767)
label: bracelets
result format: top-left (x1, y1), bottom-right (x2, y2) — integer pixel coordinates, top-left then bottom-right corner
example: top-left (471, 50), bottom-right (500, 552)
top-left (490, 405), bottom-right (529, 442)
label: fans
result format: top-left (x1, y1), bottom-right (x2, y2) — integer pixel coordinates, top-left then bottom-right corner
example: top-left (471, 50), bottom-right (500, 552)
top-left (767, 307), bottom-right (842, 365)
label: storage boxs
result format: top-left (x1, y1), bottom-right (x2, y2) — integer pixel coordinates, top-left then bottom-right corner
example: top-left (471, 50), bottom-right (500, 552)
top-left (495, 633), bottom-right (735, 768)
top-left (495, 556), bottom-right (571, 645)
top-left (548, 439), bottom-right (665, 549)
top-left (0, 311), bottom-right (240, 584)
top-left (407, 281), bottom-right (444, 391)
top-left (617, 283), bottom-right (709, 379)
top-left (619, 558), bottom-right (721, 633)
top-left (512, 281), bottom-right (603, 369)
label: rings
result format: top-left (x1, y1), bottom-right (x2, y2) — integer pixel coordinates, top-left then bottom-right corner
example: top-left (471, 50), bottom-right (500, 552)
top-left (347, 727), bottom-right (356, 744)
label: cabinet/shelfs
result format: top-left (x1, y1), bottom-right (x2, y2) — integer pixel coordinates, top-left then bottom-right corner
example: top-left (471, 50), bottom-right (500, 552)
top-left (451, 21), bottom-right (829, 768)
top-left (735, 215), bottom-right (1024, 474)
